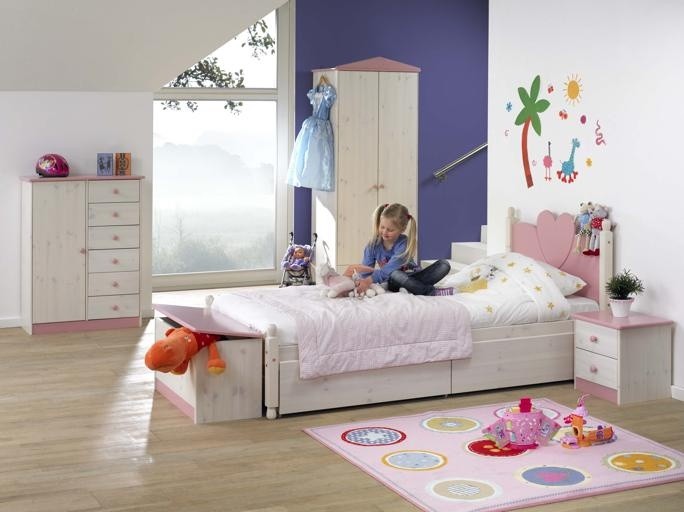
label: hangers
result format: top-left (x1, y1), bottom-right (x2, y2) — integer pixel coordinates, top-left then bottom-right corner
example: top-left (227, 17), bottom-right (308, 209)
top-left (317, 69), bottom-right (327, 88)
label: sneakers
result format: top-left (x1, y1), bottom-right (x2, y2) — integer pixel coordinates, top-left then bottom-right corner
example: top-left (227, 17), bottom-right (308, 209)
top-left (431, 287), bottom-right (457, 296)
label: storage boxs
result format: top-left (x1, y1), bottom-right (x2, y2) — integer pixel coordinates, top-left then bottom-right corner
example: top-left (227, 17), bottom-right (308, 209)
top-left (153, 303), bottom-right (265, 424)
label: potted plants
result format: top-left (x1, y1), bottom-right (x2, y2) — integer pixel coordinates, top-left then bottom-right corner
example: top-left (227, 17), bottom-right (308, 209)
top-left (604, 268), bottom-right (646, 318)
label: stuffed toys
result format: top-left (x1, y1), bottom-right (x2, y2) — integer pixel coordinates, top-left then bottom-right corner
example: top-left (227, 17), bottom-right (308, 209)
top-left (318, 257), bottom-right (385, 300)
top-left (144, 326), bottom-right (225, 377)
top-left (571, 200), bottom-right (614, 257)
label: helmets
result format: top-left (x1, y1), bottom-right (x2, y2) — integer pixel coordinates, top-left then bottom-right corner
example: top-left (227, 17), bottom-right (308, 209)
top-left (36, 153), bottom-right (69, 177)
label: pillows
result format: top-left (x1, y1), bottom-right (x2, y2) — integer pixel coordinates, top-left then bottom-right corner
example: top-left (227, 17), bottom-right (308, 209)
top-left (536, 258), bottom-right (588, 299)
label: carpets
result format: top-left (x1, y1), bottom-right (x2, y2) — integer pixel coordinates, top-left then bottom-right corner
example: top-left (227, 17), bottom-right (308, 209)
top-left (300, 396), bottom-right (684, 512)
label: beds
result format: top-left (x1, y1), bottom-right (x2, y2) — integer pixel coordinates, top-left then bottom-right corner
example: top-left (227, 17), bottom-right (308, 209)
top-left (202, 205), bottom-right (613, 420)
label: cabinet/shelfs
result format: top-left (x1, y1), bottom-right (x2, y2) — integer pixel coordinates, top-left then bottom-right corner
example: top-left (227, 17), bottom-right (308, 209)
top-left (20, 171), bottom-right (146, 338)
top-left (311, 57), bottom-right (423, 284)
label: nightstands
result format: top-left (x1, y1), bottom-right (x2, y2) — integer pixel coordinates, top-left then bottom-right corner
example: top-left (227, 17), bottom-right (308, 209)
top-left (573, 312), bottom-right (675, 409)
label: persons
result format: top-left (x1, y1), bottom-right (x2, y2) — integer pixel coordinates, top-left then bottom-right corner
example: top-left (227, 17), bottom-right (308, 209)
top-left (355, 202), bottom-right (459, 297)
top-left (279, 232), bottom-right (318, 289)
